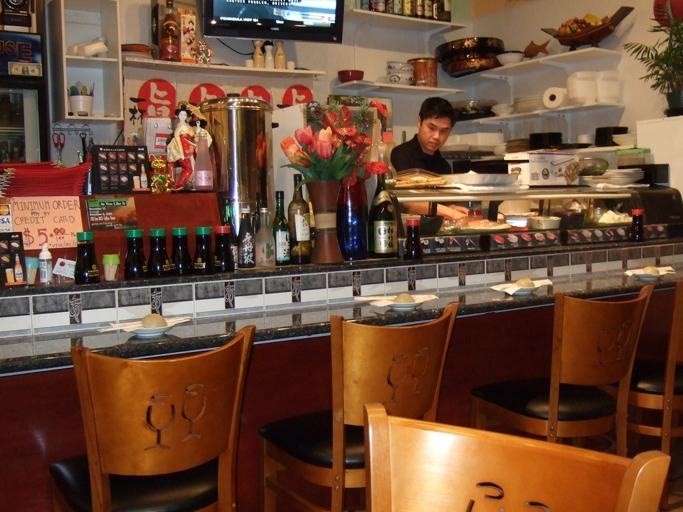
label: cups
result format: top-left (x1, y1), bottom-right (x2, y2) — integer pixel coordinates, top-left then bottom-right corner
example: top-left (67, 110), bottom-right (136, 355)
top-left (69, 94), bottom-right (94, 116)
top-left (132, 176), bottom-right (141, 190)
top-left (245, 59), bottom-right (253, 67)
top-left (24, 256), bottom-right (39, 285)
top-left (100, 254), bottom-right (121, 282)
top-left (4, 268), bottom-right (15, 284)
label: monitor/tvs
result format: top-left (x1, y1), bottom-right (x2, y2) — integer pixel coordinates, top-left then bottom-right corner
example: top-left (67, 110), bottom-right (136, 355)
top-left (203, 0), bottom-right (344, 43)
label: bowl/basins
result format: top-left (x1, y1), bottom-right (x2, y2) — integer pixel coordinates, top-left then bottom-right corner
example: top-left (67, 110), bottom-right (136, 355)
top-left (433, 36), bottom-right (504, 78)
top-left (447, 98), bottom-right (498, 121)
top-left (489, 103), bottom-right (513, 115)
top-left (553, 207), bottom-right (586, 229)
top-left (494, 50), bottom-right (524, 66)
top-left (337, 69), bottom-right (364, 84)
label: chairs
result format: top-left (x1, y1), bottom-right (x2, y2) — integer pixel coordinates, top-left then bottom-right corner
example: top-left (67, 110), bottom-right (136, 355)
top-left (260, 297), bottom-right (461, 511)
top-left (469, 283), bottom-right (655, 460)
top-left (628, 276), bottom-right (682, 467)
top-left (73, 324), bottom-right (258, 512)
top-left (362, 401), bottom-right (672, 512)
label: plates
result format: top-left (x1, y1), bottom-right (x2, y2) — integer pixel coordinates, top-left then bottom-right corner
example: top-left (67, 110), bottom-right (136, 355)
top-left (611, 133), bottom-right (636, 147)
top-left (511, 95), bottom-right (543, 114)
top-left (128, 321), bottom-right (174, 337)
top-left (548, 142), bottom-right (591, 149)
top-left (504, 287), bottom-right (538, 295)
top-left (529, 130), bottom-right (562, 150)
top-left (388, 303), bottom-right (421, 311)
top-left (575, 133), bottom-right (595, 147)
top-left (460, 223), bottom-right (511, 234)
top-left (584, 168), bottom-right (643, 188)
top-left (634, 273), bottom-right (662, 281)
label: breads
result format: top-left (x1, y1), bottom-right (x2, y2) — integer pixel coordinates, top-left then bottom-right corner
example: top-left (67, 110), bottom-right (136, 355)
top-left (143, 314), bottom-right (167, 328)
top-left (644, 266), bottom-right (659, 274)
top-left (516, 279), bottom-right (534, 287)
top-left (394, 294), bottom-right (415, 303)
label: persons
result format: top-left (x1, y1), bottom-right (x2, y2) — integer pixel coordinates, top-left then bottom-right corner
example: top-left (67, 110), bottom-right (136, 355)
top-left (391, 95), bottom-right (478, 226)
top-left (166, 103), bottom-right (213, 190)
top-left (182, 21), bottom-right (194, 36)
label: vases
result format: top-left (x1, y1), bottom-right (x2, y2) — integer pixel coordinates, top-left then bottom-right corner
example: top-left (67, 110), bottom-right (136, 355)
top-left (308, 176), bottom-right (347, 269)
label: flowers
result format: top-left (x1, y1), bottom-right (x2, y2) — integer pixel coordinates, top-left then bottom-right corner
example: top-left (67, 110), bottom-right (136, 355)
top-left (278, 95), bottom-right (387, 186)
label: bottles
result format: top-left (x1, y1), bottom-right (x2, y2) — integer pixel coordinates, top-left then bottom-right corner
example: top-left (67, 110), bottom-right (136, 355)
top-left (146, 228), bottom-right (170, 278)
top-left (211, 223), bottom-right (235, 273)
top-left (253, 206), bottom-right (277, 267)
top-left (190, 224), bottom-right (214, 276)
top-left (235, 208), bottom-right (255, 269)
top-left (271, 191), bottom-right (290, 265)
top-left (404, 218), bottom-right (424, 261)
top-left (140, 164), bottom-right (147, 189)
top-left (369, 170), bottom-right (398, 258)
top-left (335, 175), bottom-right (368, 262)
top-left (353, 0), bottom-right (452, 23)
top-left (13, 254), bottom-right (24, 283)
top-left (72, 231), bottom-right (99, 284)
top-left (123, 228), bottom-right (146, 277)
top-left (286, 173), bottom-right (311, 264)
top-left (629, 209), bottom-right (644, 242)
top-left (273, 40), bottom-right (287, 69)
top-left (467, 206), bottom-right (482, 220)
top-left (194, 130), bottom-right (213, 190)
top-left (159, 0), bottom-right (179, 62)
top-left (38, 244), bottom-right (54, 285)
top-left (168, 224), bottom-right (190, 275)
top-left (263, 43), bottom-right (274, 69)
top-left (252, 40), bottom-right (265, 68)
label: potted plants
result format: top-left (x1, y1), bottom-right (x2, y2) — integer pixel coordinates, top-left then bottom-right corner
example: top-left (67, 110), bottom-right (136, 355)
top-left (625, 1), bottom-right (683, 116)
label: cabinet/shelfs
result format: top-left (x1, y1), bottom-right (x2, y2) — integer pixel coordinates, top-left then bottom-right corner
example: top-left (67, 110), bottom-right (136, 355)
top-left (53, 1), bottom-right (124, 122)
top-left (0, 0), bottom-right (54, 162)
top-left (448, 47), bottom-right (628, 156)
top-left (335, 9), bottom-right (467, 92)
